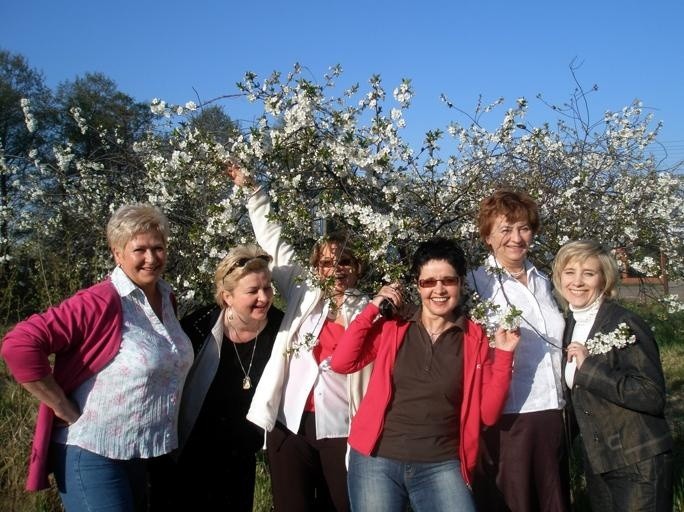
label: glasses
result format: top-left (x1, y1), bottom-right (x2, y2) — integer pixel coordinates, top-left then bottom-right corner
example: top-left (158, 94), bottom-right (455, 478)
top-left (317, 257), bottom-right (354, 268)
top-left (417, 275), bottom-right (461, 290)
top-left (222, 253), bottom-right (270, 288)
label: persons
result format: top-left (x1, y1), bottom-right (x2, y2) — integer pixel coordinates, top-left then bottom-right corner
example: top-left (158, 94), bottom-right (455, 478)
top-left (1, 161), bottom-right (676, 512)
